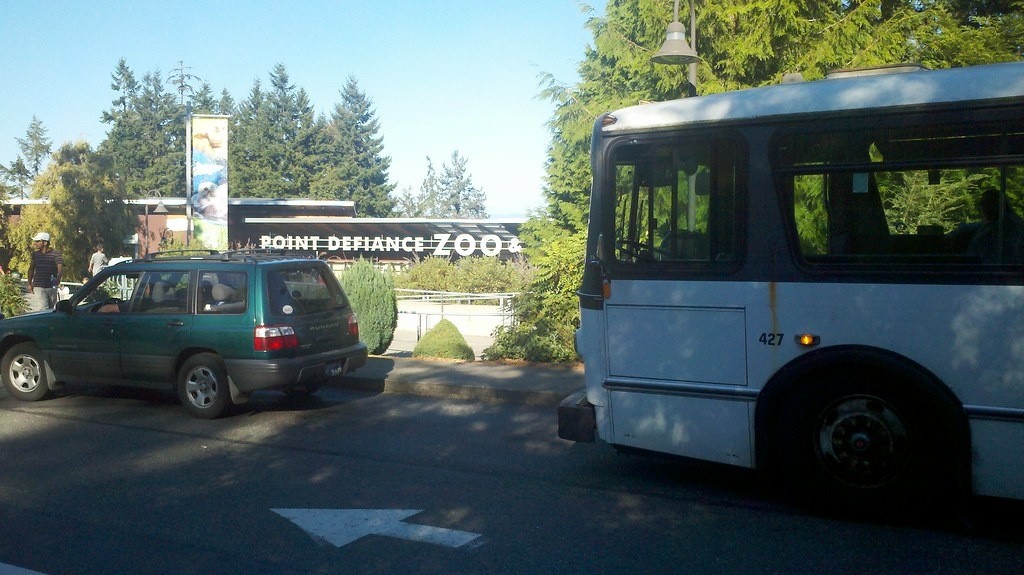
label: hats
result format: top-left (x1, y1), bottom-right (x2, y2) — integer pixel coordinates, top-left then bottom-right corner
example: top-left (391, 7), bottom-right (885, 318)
top-left (32, 232), bottom-right (50, 242)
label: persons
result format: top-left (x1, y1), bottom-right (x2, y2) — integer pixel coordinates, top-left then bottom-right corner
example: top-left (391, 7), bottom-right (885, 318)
top-left (943, 190), bottom-right (1024, 263)
top-left (88, 244), bottom-right (108, 277)
top-left (99, 274), bottom-right (160, 313)
top-left (27, 232), bottom-right (65, 312)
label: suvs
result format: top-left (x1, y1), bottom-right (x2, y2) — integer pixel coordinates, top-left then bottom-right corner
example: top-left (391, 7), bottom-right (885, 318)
top-left (0, 247), bottom-right (369, 421)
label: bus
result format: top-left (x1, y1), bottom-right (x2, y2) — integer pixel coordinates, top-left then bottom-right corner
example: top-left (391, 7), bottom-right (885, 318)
top-left (556, 62), bottom-right (1024, 534)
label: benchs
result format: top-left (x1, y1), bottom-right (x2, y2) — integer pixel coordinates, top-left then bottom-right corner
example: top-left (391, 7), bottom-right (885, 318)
top-left (207, 284), bottom-right (247, 313)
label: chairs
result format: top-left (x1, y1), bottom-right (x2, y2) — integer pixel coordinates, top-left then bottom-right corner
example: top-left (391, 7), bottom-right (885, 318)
top-left (825, 155), bottom-right (898, 256)
top-left (147, 280), bottom-right (180, 315)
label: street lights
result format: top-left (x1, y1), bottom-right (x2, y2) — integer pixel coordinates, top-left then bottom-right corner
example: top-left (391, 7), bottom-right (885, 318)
top-left (649, 0), bottom-right (707, 260)
top-left (144, 189), bottom-right (171, 256)
